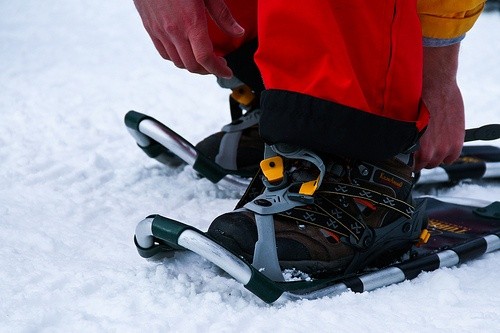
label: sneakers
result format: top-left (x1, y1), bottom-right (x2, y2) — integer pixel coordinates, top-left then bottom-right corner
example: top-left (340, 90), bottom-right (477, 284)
top-left (198, 90), bottom-right (265, 173)
top-left (209, 145), bottom-right (422, 277)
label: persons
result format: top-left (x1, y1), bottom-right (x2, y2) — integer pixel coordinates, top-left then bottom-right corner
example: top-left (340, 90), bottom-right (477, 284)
top-left (131, 0), bottom-right (486, 277)
